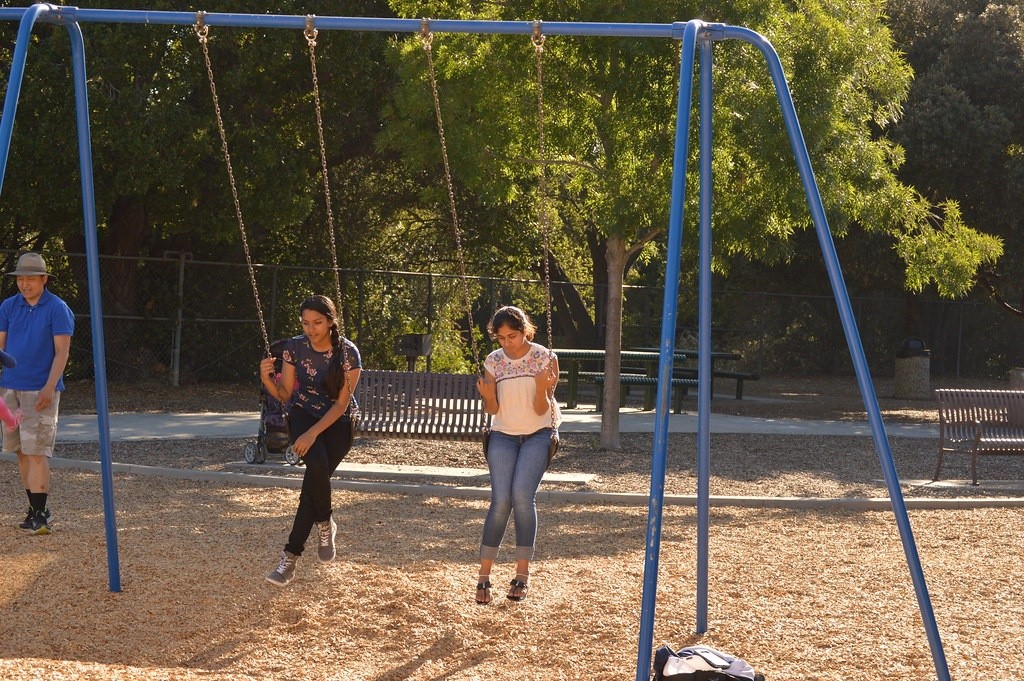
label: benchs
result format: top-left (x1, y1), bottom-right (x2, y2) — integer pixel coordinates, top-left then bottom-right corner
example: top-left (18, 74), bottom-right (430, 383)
top-left (559, 367), bottom-right (760, 412)
top-left (932, 389), bottom-right (1024, 486)
top-left (355, 369), bottom-right (490, 442)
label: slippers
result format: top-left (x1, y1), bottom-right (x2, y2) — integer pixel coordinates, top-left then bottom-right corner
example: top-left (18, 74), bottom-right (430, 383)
top-left (506, 579), bottom-right (529, 601)
top-left (475, 581), bottom-right (491, 604)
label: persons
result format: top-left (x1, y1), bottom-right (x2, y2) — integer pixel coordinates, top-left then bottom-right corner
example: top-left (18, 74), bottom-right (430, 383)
top-left (0, 253), bottom-right (75, 534)
top-left (475, 306), bottom-right (564, 603)
top-left (260, 295), bottom-right (362, 587)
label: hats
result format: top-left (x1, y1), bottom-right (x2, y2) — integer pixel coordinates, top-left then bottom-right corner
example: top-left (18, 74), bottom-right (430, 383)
top-left (6, 253), bottom-right (57, 281)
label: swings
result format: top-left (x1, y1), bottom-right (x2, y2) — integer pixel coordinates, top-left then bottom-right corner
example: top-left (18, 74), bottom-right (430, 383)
top-left (413, 29), bottom-right (566, 467)
top-left (190, 24), bottom-right (361, 460)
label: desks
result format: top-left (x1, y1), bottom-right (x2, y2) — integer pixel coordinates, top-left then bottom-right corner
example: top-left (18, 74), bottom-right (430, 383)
top-left (552, 347), bottom-right (742, 413)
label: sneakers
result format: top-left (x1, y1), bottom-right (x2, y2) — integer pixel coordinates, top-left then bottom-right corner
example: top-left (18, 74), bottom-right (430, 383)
top-left (314, 514), bottom-right (337, 564)
top-left (14, 505), bottom-right (54, 533)
top-left (28, 506), bottom-right (52, 535)
top-left (265, 548), bottom-right (298, 587)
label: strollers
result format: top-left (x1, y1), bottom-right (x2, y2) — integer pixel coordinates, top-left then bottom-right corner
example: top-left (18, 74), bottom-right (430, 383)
top-left (244, 382), bottom-right (305, 466)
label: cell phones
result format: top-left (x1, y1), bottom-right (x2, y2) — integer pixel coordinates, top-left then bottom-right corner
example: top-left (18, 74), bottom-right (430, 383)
top-left (694, 649), bottom-right (731, 669)
